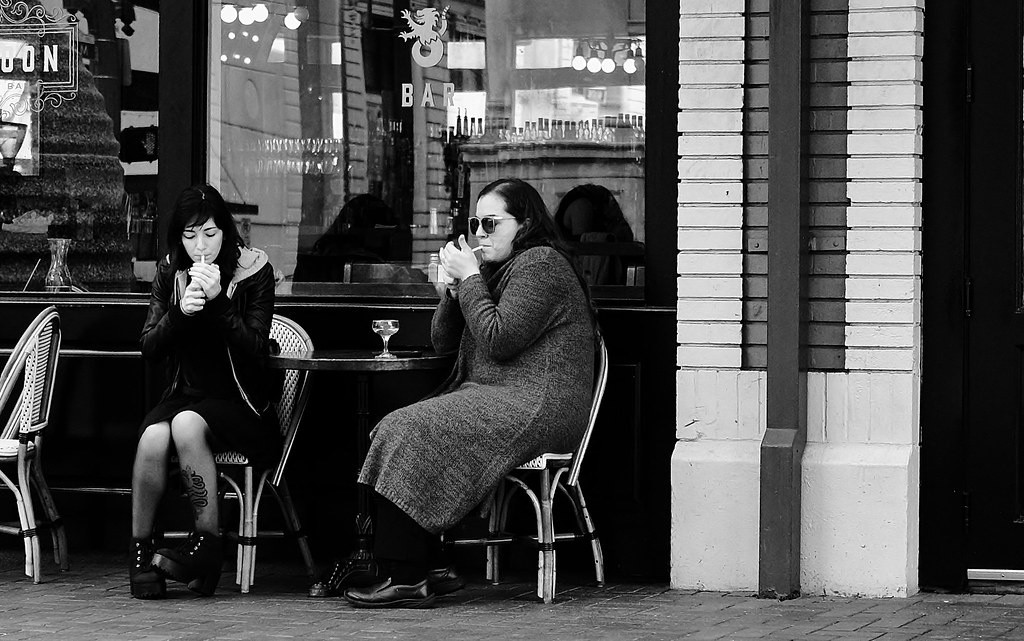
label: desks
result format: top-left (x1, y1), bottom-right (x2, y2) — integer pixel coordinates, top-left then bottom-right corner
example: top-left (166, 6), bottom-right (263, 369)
top-left (265, 351), bottom-right (456, 597)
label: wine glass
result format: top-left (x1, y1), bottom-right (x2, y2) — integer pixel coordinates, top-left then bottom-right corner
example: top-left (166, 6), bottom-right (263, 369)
top-left (372, 320), bottom-right (399, 359)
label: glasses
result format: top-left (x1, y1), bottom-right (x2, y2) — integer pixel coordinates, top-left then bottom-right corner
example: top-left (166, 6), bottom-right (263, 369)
top-left (468, 216), bottom-right (515, 236)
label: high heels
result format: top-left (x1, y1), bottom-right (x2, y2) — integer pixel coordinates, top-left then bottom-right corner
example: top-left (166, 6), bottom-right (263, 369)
top-left (129, 532), bottom-right (166, 600)
top-left (152, 530), bottom-right (225, 597)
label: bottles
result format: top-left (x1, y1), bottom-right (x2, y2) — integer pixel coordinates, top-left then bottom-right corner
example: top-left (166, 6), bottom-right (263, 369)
top-left (537, 118), bottom-right (543, 150)
top-left (523, 120), bottom-right (530, 151)
top-left (570, 121), bottom-right (576, 149)
top-left (550, 120), bottom-right (557, 149)
top-left (578, 121), bottom-right (583, 139)
top-left (543, 119), bottom-right (549, 150)
top-left (530, 122), bottom-right (536, 151)
top-left (583, 120), bottom-right (590, 140)
top-left (592, 113), bottom-right (645, 153)
top-left (449, 115), bottom-right (523, 155)
top-left (563, 121), bottom-right (570, 149)
top-left (428, 254), bottom-right (439, 282)
top-left (557, 120), bottom-right (563, 149)
top-left (45, 238), bottom-right (72, 292)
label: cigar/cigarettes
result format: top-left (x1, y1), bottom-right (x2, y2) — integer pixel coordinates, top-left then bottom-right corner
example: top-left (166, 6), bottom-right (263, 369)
top-left (472, 245), bottom-right (483, 252)
top-left (200, 255), bottom-right (205, 263)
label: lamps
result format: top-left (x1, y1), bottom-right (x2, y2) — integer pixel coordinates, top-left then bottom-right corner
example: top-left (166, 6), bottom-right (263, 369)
top-left (571, 28), bottom-right (645, 74)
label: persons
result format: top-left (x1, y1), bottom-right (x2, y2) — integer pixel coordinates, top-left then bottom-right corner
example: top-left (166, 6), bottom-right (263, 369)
top-left (294, 194), bottom-right (413, 282)
top-left (129, 184), bottom-right (276, 600)
top-left (309, 177), bottom-right (594, 608)
top-left (555, 184), bottom-right (646, 286)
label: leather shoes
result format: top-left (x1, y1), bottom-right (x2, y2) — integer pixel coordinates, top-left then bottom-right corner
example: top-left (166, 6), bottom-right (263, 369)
top-left (343, 577), bottom-right (436, 609)
top-left (425, 564), bottom-right (463, 597)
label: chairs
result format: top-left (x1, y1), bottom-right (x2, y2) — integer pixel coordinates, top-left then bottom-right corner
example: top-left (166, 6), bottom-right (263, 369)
top-left (0, 305), bottom-right (68, 584)
top-left (163, 313), bottom-right (315, 593)
top-left (449, 328), bottom-right (610, 602)
